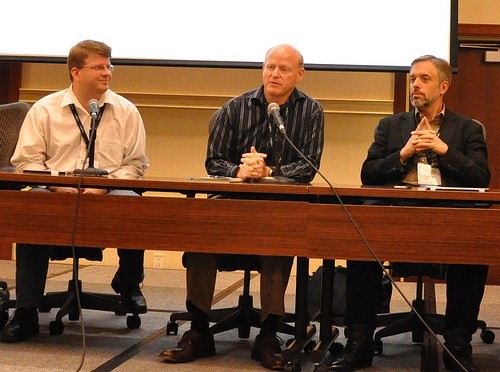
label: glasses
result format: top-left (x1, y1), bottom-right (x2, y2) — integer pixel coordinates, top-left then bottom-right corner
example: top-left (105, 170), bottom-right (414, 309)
top-left (76, 64), bottom-right (115, 73)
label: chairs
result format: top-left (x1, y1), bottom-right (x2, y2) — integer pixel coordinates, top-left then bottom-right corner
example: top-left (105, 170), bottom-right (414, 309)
top-left (373, 118), bottom-right (496, 355)
top-left (0, 102), bottom-right (31, 168)
top-left (166, 108), bottom-right (296, 345)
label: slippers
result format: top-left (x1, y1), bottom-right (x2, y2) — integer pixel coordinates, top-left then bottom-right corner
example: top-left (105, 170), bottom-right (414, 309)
top-left (328, 323), bottom-right (375, 368)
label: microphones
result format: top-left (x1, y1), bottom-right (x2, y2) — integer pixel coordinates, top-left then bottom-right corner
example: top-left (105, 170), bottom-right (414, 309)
top-left (268, 103), bottom-right (286, 135)
top-left (87, 99), bottom-right (99, 119)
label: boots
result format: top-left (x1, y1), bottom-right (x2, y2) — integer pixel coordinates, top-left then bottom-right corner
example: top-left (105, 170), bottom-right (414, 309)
top-left (442, 327), bottom-right (481, 372)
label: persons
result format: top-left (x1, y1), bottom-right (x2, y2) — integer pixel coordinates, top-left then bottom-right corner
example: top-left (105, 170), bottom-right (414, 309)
top-left (0, 41), bottom-right (151, 343)
top-left (160, 44), bottom-right (324, 372)
top-left (330, 55), bottom-right (490, 370)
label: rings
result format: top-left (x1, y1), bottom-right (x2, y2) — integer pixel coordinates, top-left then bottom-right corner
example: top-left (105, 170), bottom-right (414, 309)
top-left (416, 135), bottom-right (419, 139)
top-left (254, 168), bottom-right (257, 172)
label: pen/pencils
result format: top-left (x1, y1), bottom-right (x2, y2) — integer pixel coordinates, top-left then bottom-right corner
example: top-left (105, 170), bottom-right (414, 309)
top-left (208, 175), bottom-right (224, 178)
top-left (393, 185), bottom-right (411, 189)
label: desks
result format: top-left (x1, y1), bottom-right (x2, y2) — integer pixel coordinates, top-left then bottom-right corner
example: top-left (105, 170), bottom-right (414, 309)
top-left (0, 171), bottom-right (500, 265)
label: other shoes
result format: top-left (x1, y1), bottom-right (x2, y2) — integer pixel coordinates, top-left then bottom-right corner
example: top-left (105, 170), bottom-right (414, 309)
top-left (0, 313), bottom-right (40, 343)
top-left (111, 275), bottom-right (147, 313)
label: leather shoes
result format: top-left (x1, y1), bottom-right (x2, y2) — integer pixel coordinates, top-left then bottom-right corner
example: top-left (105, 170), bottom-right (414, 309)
top-left (252, 333), bottom-right (286, 369)
top-left (160, 329), bottom-right (216, 362)
